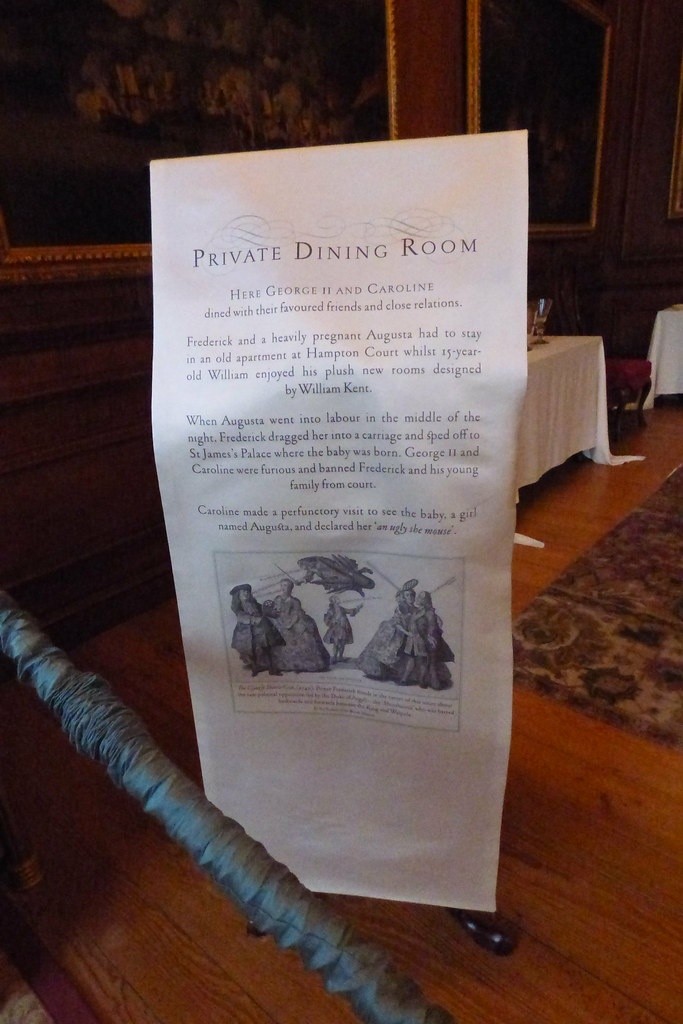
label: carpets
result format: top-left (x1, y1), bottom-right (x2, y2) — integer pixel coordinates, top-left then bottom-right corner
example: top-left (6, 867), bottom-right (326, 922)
top-left (512, 462), bottom-right (683, 747)
top-left (0, 893), bottom-right (112, 1024)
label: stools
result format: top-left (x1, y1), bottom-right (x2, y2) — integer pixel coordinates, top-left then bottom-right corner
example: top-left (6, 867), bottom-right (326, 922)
top-left (606, 360), bottom-right (653, 430)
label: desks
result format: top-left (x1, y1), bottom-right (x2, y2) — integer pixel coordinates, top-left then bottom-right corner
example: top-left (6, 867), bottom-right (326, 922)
top-left (643, 304), bottom-right (683, 410)
top-left (513, 336), bottom-right (645, 548)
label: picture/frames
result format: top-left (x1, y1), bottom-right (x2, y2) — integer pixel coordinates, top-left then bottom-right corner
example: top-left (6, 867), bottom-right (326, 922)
top-left (666, 64), bottom-right (683, 218)
top-left (466, 0), bottom-right (612, 239)
top-left (0, 1), bottom-right (398, 285)
